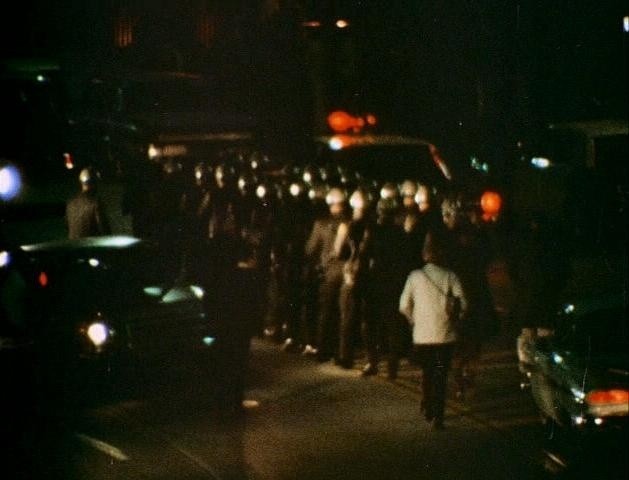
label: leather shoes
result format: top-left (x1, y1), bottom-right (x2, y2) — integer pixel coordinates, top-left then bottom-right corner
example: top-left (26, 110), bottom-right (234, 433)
top-left (264, 330), bottom-right (396, 380)
top-left (420, 398), bottom-right (433, 424)
top-left (431, 418), bottom-right (443, 430)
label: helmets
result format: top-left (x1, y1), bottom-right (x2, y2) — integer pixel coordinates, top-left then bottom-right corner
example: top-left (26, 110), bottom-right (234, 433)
top-left (164, 141), bottom-right (464, 217)
top-left (79, 166), bottom-right (104, 184)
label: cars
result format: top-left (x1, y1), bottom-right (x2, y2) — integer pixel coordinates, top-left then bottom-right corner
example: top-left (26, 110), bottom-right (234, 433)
top-left (0, 235), bottom-right (253, 411)
top-left (516, 283), bottom-right (629, 443)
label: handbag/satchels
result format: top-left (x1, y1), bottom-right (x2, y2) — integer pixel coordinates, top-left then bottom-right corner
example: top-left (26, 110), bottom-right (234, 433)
top-left (446, 296), bottom-right (472, 336)
